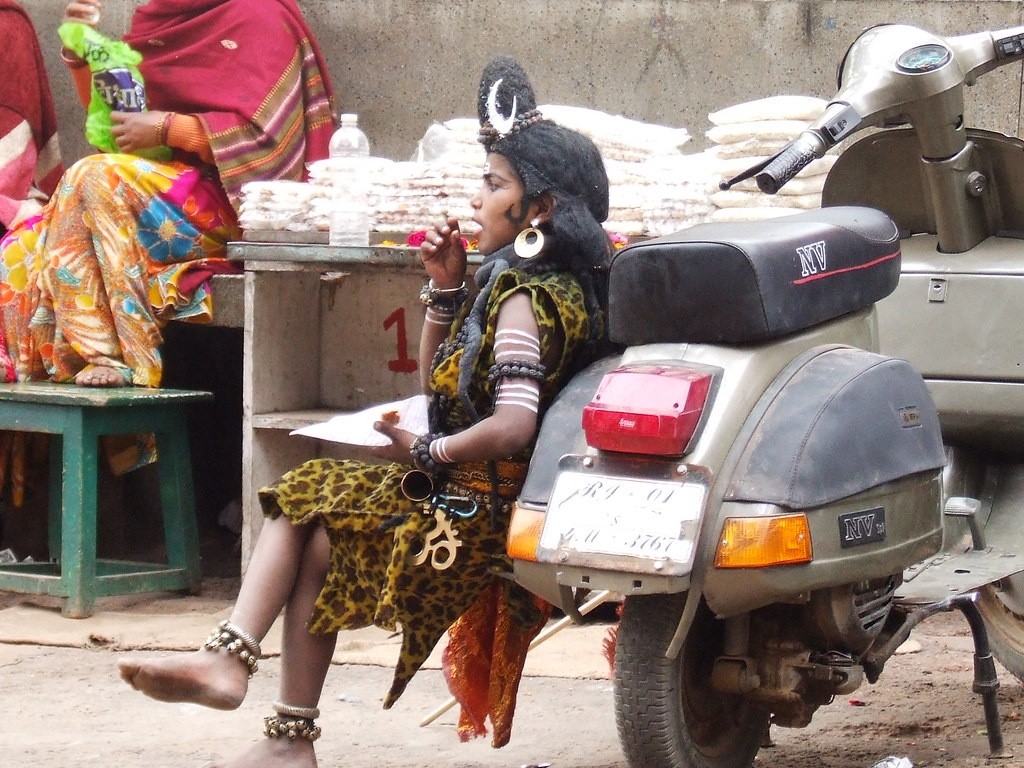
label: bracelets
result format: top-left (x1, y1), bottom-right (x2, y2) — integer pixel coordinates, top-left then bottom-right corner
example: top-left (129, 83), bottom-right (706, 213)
top-left (155, 110), bottom-right (175, 145)
top-left (59, 45), bottom-right (89, 68)
top-left (411, 432), bottom-right (445, 476)
top-left (420, 279), bottom-right (468, 313)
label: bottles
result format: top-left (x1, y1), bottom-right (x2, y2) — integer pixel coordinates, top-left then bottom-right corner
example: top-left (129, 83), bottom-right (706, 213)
top-left (327, 113), bottom-right (370, 247)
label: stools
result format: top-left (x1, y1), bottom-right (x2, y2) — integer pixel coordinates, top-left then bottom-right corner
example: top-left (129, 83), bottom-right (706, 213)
top-left (1, 380), bottom-right (214, 618)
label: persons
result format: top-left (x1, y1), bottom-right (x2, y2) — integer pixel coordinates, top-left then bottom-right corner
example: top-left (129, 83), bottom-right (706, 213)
top-left (0, 0), bottom-right (337, 386)
top-left (117, 60), bottom-right (621, 768)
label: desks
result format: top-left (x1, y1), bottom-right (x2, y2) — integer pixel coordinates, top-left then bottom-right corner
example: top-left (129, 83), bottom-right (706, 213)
top-left (226, 242), bottom-right (485, 591)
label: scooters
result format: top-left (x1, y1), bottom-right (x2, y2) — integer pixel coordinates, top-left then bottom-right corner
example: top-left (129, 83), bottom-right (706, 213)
top-left (506, 18), bottom-right (1024, 768)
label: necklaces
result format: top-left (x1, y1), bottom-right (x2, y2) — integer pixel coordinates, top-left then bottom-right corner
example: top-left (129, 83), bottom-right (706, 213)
top-left (428, 312), bottom-right (469, 376)
